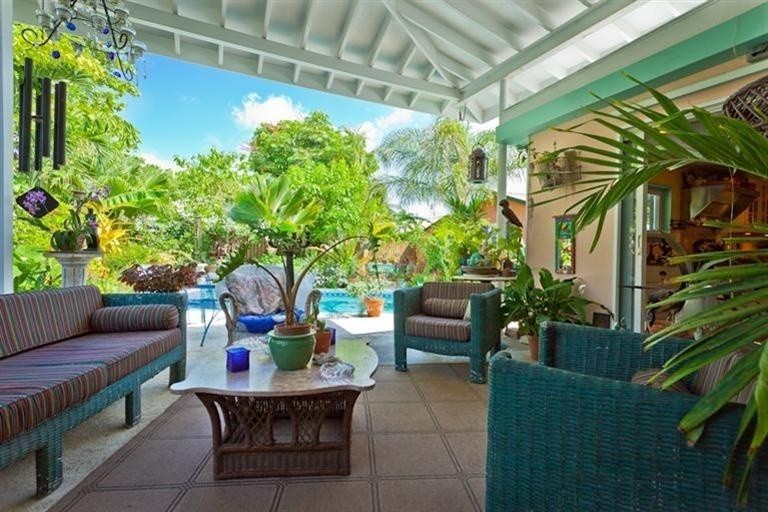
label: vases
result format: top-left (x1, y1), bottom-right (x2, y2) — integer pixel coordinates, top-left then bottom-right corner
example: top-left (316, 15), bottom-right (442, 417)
top-left (53, 230), bottom-right (85, 254)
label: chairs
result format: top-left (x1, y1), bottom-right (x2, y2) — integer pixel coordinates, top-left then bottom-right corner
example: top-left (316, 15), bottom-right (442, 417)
top-left (484, 323), bottom-right (766, 511)
top-left (392, 282), bottom-right (503, 384)
top-left (219, 264), bottom-right (323, 351)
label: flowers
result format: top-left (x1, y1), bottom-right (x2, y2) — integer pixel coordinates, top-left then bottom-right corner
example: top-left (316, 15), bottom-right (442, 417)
top-left (22, 184), bottom-right (111, 254)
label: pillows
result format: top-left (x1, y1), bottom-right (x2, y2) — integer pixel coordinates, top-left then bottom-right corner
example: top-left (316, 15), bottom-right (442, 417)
top-left (422, 297), bottom-right (471, 319)
top-left (90, 304), bottom-right (180, 331)
top-left (631, 369), bottom-right (690, 392)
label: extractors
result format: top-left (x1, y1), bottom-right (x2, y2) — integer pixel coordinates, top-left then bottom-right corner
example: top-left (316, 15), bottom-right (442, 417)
top-left (680, 184), bottom-right (761, 229)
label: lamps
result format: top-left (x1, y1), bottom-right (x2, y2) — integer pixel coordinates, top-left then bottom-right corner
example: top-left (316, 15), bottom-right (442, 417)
top-left (466, 109), bottom-right (489, 184)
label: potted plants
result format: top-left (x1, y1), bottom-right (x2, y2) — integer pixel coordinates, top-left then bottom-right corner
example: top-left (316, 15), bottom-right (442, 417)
top-left (214, 223), bottom-right (402, 371)
top-left (499, 263), bottom-right (615, 363)
top-left (346, 286), bottom-right (384, 317)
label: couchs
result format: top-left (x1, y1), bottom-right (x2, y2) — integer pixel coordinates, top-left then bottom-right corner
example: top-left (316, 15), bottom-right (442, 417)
top-left (0, 283), bottom-right (187, 494)
